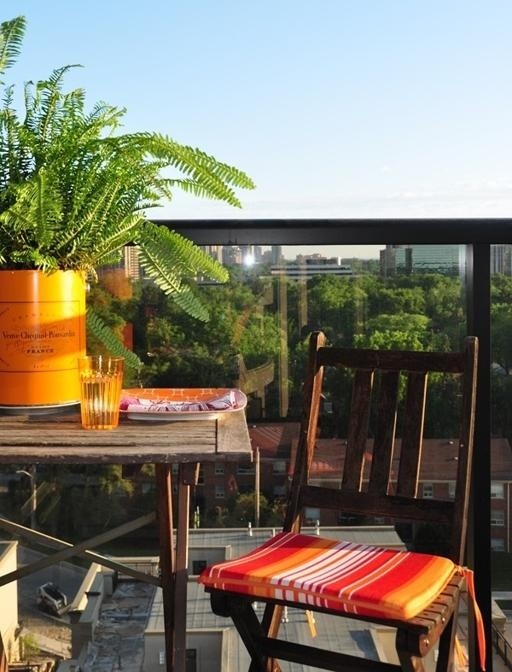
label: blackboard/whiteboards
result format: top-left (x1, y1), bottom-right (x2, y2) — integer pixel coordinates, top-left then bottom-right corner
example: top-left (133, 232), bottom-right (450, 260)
top-left (39, 582), bottom-right (71, 616)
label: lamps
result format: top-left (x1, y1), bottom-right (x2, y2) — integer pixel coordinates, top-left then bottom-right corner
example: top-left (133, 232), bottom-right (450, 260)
top-left (201, 332), bottom-right (479, 672)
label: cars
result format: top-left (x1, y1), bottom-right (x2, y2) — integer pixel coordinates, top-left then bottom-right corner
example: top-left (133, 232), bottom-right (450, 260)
top-left (77, 354), bottom-right (125, 430)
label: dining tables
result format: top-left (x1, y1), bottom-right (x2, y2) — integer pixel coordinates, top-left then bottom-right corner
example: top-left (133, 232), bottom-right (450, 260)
top-left (0, 15), bottom-right (254, 406)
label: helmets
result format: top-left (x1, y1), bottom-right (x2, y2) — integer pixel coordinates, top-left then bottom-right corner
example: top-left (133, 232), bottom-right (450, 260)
top-left (118, 387), bottom-right (248, 421)
